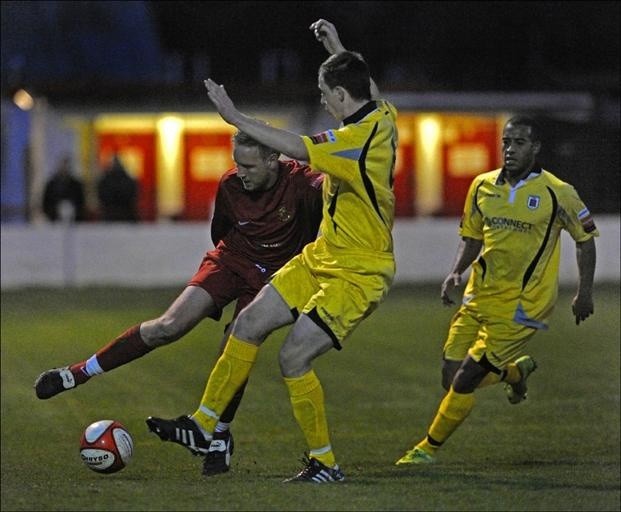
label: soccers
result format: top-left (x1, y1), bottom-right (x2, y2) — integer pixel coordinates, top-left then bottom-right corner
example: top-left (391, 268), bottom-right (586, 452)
top-left (80, 419), bottom-right (134, 474)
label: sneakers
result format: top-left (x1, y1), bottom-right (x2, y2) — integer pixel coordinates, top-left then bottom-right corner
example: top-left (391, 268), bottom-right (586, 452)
top-left (145, 413), bottom-right (213, 457)
top-left (200, 432), bottom-right (234, 476)
top-left (503, 355), bottom-right (538, 405)
top-left (281, 450), bottom-right (345, 484)
top-left (394, 445), bottom-right (440, 466)
top-left (35, 365), bottom-right (78, 400)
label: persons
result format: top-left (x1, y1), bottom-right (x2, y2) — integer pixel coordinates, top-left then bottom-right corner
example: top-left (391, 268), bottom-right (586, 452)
top-left (95, 154), bottom-right (140, 224)
top-left (34, 130), bottom-right (324, 475)
top-left (395, 115), bottom-right (599, 465)
top-left (146, 20), bottom-right (398, 483)
top-left (42, 153), bottom-right (87, 223)
